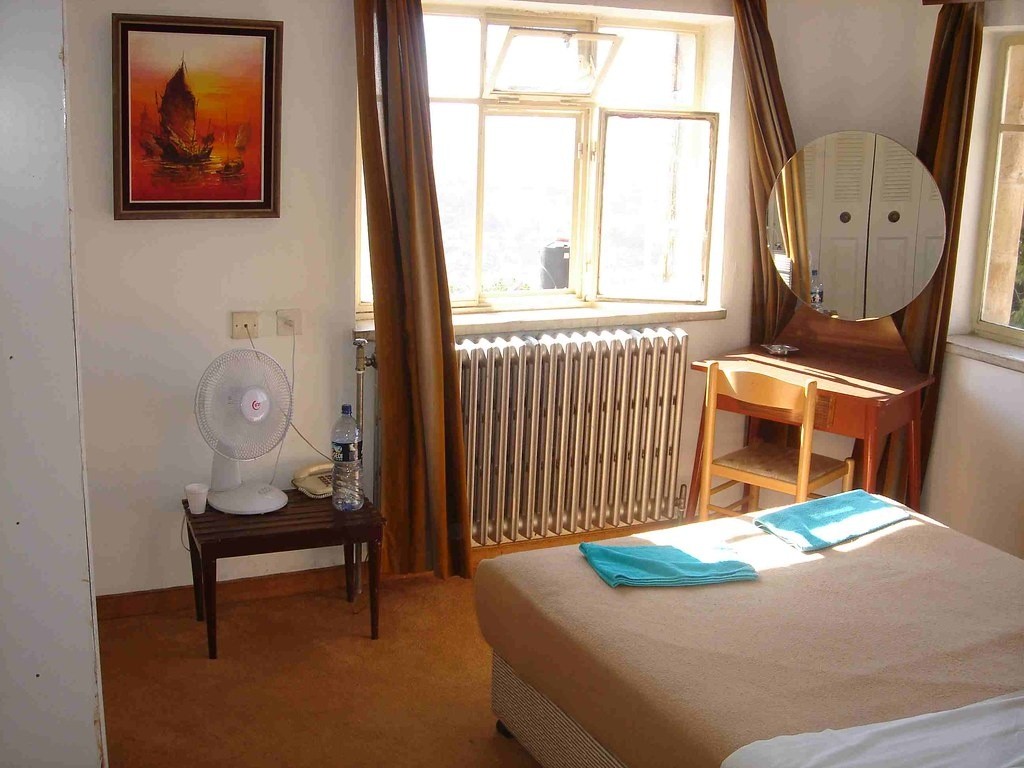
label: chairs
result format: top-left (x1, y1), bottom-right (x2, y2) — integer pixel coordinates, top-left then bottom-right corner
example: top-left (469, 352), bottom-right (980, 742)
top-left (696, 359), bottom-right (856, 522)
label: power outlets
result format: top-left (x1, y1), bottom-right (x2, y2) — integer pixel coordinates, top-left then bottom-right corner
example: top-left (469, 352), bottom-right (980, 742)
top-left (277, 309), bottom-right (302, 337)
top-left (231, 312), bottom-right (259, 339)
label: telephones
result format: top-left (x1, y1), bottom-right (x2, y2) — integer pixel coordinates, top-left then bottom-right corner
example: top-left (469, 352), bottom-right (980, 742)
top-left (290, 462), bottom-right (335, 499)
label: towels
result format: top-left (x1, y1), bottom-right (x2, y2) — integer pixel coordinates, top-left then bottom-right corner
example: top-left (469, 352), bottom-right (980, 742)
top-left (578, 541), bottom-right (759, 589)
top-left (752, 488), bottom-right (912, 552)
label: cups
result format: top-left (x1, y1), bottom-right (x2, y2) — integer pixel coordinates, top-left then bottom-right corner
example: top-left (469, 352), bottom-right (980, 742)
top-left (184, 483), bottom-right (209, 514)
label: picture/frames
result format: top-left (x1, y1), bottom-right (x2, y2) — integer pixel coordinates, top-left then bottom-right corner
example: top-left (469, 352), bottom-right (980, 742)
top-left (111, 11), bottom-right (283, 219)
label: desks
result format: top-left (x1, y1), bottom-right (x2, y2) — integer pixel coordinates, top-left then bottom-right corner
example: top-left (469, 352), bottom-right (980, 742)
top-left (688, 344), bottom-right (936, 515)
top-left (179, 490), bottom-right (386, 660)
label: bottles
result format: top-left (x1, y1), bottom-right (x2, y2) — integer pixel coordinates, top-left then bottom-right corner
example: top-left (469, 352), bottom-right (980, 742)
top-left (332, 404), bottom-right (364, 511)
top-left (811, 270), bottom-right (823, 313)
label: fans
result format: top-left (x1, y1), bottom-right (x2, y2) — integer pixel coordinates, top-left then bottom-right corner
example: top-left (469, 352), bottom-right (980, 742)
top-left (192, 346), bottom-right (294, 515)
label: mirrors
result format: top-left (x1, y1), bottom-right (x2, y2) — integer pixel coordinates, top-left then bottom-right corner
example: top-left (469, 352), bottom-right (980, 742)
top-left (765, 128), bottom-right (945, 350)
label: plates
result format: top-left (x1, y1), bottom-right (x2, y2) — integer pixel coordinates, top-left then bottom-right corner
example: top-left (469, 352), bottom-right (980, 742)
top-left (763, 345), bottom-right (799, 355)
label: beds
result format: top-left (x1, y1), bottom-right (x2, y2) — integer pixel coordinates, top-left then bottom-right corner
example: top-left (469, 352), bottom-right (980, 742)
top-left (472, 488), bottom-right (1023, 768)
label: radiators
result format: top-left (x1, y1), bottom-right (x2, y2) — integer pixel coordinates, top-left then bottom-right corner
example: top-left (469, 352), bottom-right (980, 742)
top-left (450, 326), bottom-right (687, 546)
